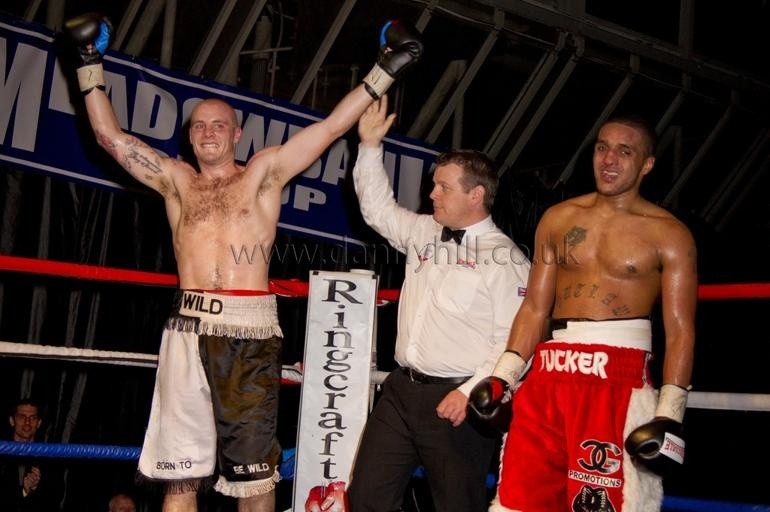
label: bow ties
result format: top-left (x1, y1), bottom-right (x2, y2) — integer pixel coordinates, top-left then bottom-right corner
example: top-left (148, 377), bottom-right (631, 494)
top-left (441, 227), bottom-right (466, 245)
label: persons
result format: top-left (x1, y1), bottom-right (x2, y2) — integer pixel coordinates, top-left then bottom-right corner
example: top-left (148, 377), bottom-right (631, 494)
top-left (467, 116), bottom-right (698, 512)
top-left (108, 494), bottom-right (136, 512)
top-left (0, 400), bottom-right (52, 512)
top-left (1, 400), bottom-right (45, 512)
top-left (59, 15), bottom-right (420, 511)
top-left (347, 95), bottom-right (535, 512)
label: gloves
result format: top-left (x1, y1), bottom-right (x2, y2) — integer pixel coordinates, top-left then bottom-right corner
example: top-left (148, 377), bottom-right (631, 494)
top-left (468, 350), bottom-right (528, 440)
top-left (362, 20), bottom-right (423, 101)
top-left (624, 384), bottom-right (695, 474)
top-left (60, 16), bottom-right (112, 94)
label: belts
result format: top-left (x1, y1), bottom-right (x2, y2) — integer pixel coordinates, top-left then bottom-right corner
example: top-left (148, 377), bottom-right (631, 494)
top-left (401, 366), bottom-right (472, 385)
